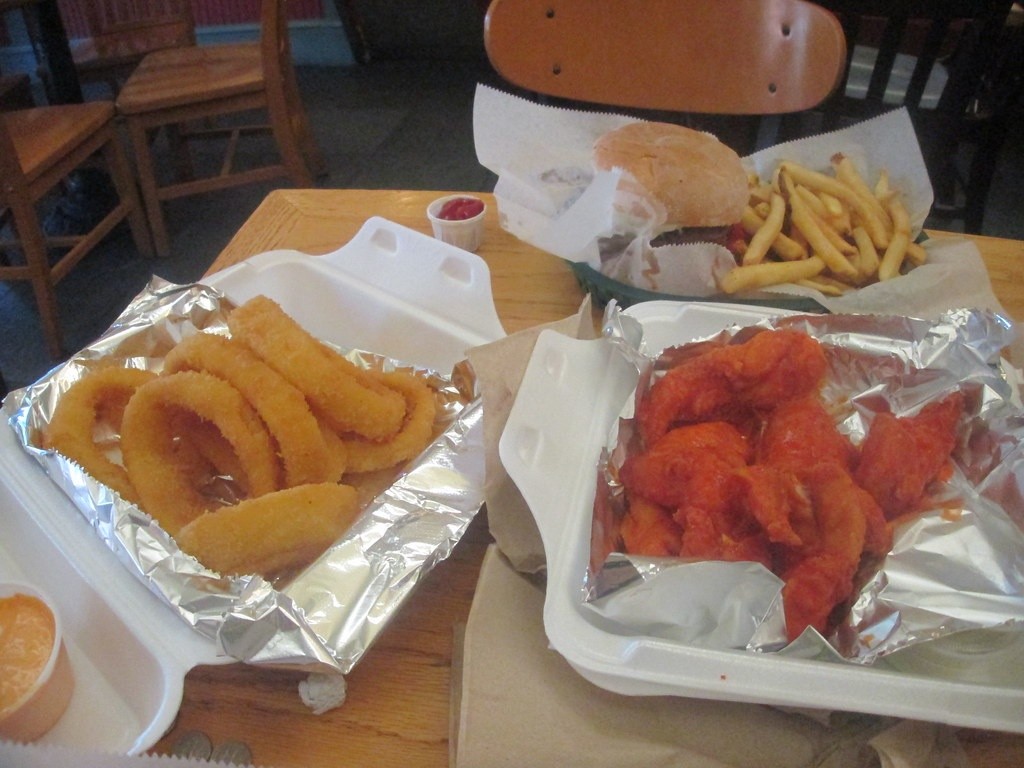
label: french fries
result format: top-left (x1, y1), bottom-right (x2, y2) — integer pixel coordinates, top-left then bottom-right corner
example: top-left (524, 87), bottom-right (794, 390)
top-left (721, 153), bottom-right (931, 295)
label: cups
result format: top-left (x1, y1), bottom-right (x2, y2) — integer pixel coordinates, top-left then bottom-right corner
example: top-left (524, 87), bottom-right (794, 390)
top-left (427, 195), bottom-right (486, 250)
top-left (0, 581), bottom-right (74, 742)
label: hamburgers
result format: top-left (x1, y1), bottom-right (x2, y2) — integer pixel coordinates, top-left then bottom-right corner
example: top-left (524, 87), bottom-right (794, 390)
top-left (588, 122), bottom-right (752, 267)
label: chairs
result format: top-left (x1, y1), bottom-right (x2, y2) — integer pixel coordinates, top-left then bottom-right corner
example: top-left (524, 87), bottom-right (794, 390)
top-left (0, 96), bottom-right (155, 366)
top-left (746, 0), bottom-right (1024, 236)
top-left (83, 0), bottom-right (850, 261)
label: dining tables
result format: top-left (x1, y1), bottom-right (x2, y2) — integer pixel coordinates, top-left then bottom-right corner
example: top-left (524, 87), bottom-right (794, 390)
top-left (139, 184), bottom-right (1023, 768)
